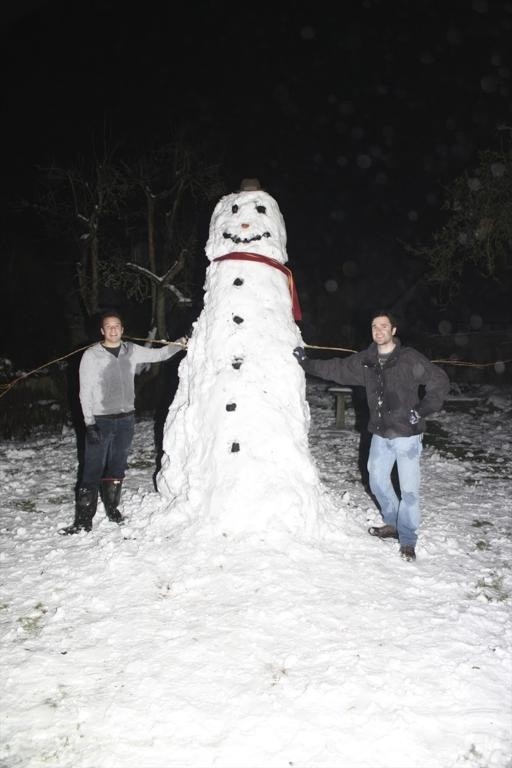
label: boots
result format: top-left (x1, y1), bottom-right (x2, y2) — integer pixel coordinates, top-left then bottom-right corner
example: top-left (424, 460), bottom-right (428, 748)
top-left (99, 477), bottom-right (129, 522)
top-left (57, 487), bottom-right (98, 535)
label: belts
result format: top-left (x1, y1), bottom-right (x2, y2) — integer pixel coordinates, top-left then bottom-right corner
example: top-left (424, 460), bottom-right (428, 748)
top-left (94, 410), bottom-right (135, 419)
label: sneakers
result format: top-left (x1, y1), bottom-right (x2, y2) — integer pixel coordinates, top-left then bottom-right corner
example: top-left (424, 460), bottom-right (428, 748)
top-left (368, 525), bottom-right (399, 539)
top-left (399, 544), bottom-right (416, 562)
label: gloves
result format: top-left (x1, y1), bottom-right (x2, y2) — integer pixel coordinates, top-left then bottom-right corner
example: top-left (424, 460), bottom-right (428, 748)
top-left (409, 408), bottom-right (421, 425)
top-left (85, 424), bottom-right (102, 445)
top-left (292, 346), bottom-right (308, 363)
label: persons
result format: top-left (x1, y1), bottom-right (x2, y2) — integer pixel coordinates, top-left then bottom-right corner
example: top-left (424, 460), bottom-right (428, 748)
top-left (292, 312), bottom-right (450, 562)
top-left (57, 312), bottom-right (187, 536)
